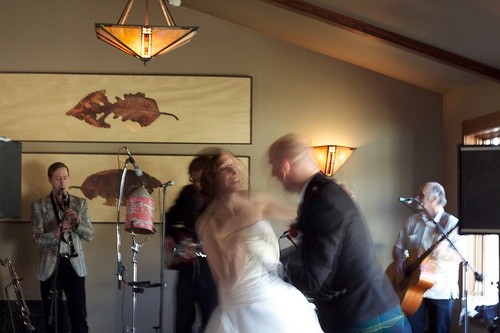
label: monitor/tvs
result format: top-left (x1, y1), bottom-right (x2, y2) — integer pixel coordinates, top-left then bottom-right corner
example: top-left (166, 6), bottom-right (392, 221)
top-left (457, 144), bottom-right (500, 234)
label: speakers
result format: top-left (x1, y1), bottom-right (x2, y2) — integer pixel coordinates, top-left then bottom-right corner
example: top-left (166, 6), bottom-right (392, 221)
top-left (0, 141), bottom-right (22, 221)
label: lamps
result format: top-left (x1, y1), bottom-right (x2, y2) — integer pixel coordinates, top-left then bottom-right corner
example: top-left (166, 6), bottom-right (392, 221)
top-left (313, 145), bottom-right (356, 177)
top-left (95, 0), bottom-right (200, 66)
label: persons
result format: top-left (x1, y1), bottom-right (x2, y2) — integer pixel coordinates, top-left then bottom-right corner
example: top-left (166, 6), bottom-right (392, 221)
top-left (391, 182), bottom-right (467, 333)
top-left (187, 145), bottom-right (324, 333)
top-left (30, 162), bottom-right (95, 333)
top-left (165, 159), bottom-right (218, 333)
top-left (268, 132), bottom-right (411, 333)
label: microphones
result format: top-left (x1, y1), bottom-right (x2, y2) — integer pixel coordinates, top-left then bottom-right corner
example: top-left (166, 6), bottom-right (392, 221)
top-left (399, 196), bottom-right (414, 204)
top-left (124, 146), bottom-right (143, 177)
top-left (159, 180), bottom-right (175, 188)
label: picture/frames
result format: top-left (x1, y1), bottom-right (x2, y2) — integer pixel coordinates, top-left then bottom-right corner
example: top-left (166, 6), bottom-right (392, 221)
top-left (0, 71), bottom-right (253, 146)
top-left (0, 151), bottom-right (250, 225)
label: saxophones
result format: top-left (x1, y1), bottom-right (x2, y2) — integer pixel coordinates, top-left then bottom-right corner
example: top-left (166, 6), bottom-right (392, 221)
top-left (62, 187), bottom-right (78, 257)
top-left (1, 259), bottom-right (35, 331)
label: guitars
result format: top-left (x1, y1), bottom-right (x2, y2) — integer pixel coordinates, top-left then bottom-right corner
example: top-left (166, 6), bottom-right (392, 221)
top-left (384, 216), bottom-right (462, 316)
top-left (164, 236), bottom-right (206, 270)
